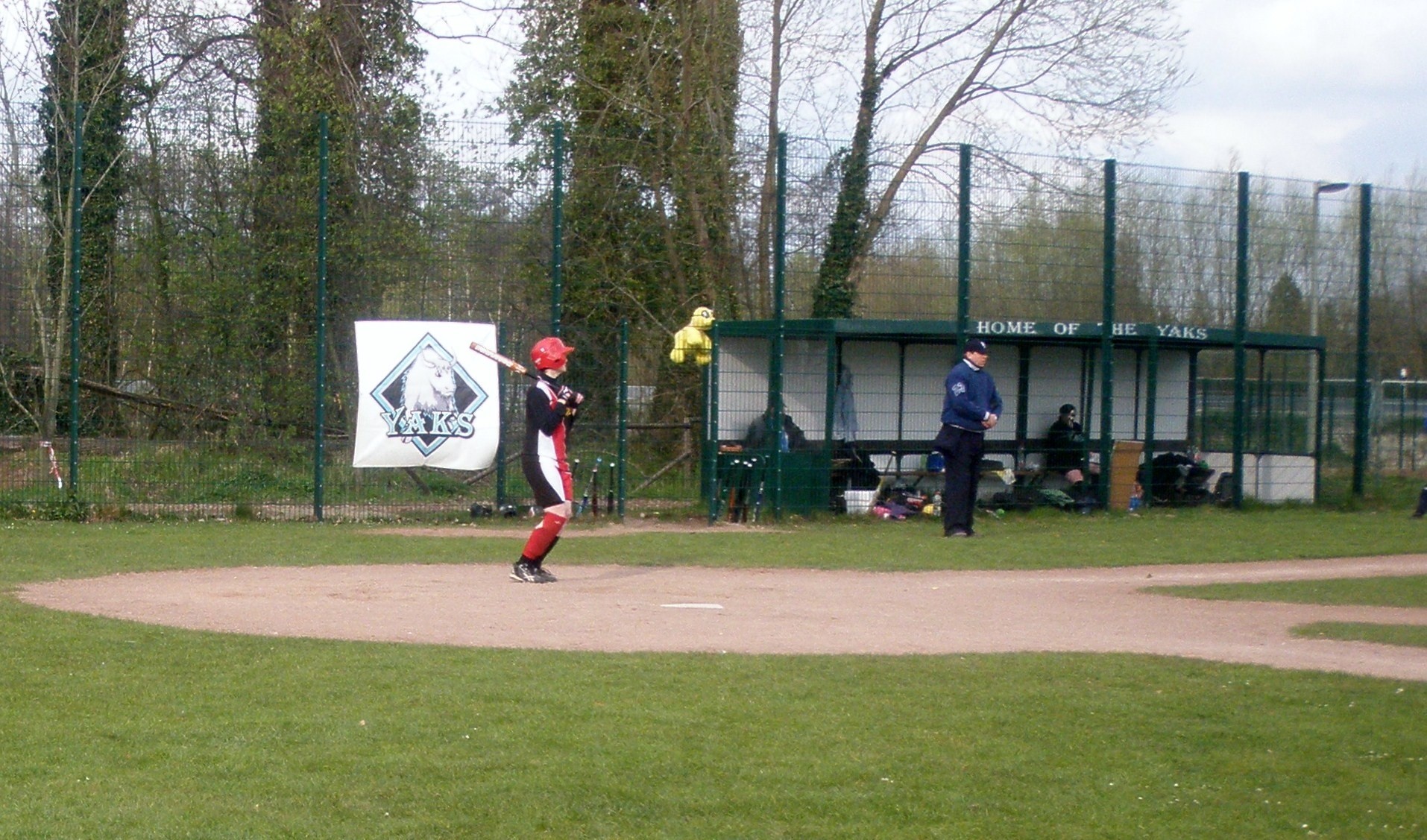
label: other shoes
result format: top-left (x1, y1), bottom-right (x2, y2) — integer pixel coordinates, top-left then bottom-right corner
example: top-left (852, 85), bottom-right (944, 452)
top-left (945, 528), bottom-right (978, 537)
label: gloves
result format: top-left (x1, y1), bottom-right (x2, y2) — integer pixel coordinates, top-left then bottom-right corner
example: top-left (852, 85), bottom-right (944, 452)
top-left (569, 392), bottom-right (584, 408)
top-left (557, 385), bottom-right (573, 405)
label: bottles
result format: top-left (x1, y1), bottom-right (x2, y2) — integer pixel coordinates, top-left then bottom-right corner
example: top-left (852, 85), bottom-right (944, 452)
top-left (1186, 445), bottom-right (1201, 464)
top-left (933, 491), bottom-right (941, 516)
top-left (781, 432), bottom-right (788, 450)
top-left (1017, 453), bottom-right (1025, 471)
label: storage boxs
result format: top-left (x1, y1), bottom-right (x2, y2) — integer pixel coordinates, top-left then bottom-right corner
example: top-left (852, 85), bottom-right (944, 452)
top-left (927, 451), bottom-right (944, 472)
top-left (845, 490), bottom-right (876, 514)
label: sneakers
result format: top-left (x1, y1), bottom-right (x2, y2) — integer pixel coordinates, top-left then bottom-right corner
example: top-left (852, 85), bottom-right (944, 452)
top-left (509, 562), bottom-right (557, 584)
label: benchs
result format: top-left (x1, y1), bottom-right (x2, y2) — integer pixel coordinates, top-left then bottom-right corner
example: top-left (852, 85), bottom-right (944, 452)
top-left (878, 468), bottom-right (1043, 475)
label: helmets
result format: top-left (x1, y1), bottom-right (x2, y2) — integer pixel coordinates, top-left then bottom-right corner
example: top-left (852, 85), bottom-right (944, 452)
top-left (530, 336), bottom-right (575, 370)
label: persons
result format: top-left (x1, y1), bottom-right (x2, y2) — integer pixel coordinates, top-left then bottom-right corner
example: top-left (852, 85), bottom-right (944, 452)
top-left (1044, 404), bottom-right (1101, 512)
top-left (744, 401), bottom-right (806, 450)
top-left (940, 338), bottom-right (1003, 538)
top-left (509, 337), bottom-right (584, 584)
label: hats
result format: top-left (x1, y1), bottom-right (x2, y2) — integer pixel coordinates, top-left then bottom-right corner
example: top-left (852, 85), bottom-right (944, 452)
top-left (966, 338), bottom-right (989, 354)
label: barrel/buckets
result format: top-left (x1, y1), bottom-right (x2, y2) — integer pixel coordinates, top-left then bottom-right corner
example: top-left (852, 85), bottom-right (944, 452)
top-left (844, 491), bottom-right (876, 515)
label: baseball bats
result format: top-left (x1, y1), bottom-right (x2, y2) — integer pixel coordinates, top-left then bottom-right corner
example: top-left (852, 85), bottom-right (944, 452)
top-left (469, 341), bottom-right (586, 406)
top-left (865, 449), bottom-right (897, 515)
top-left (592, 470), bottom-right (599, 517)
top-left (576, 458), bottom-right (603, 518)
top-left (715, 450), bottom-right (770, 523)
top-left (607, 463), bottom-right (615, 515)
top-left (570, 459), bottom-right (579, 479)
